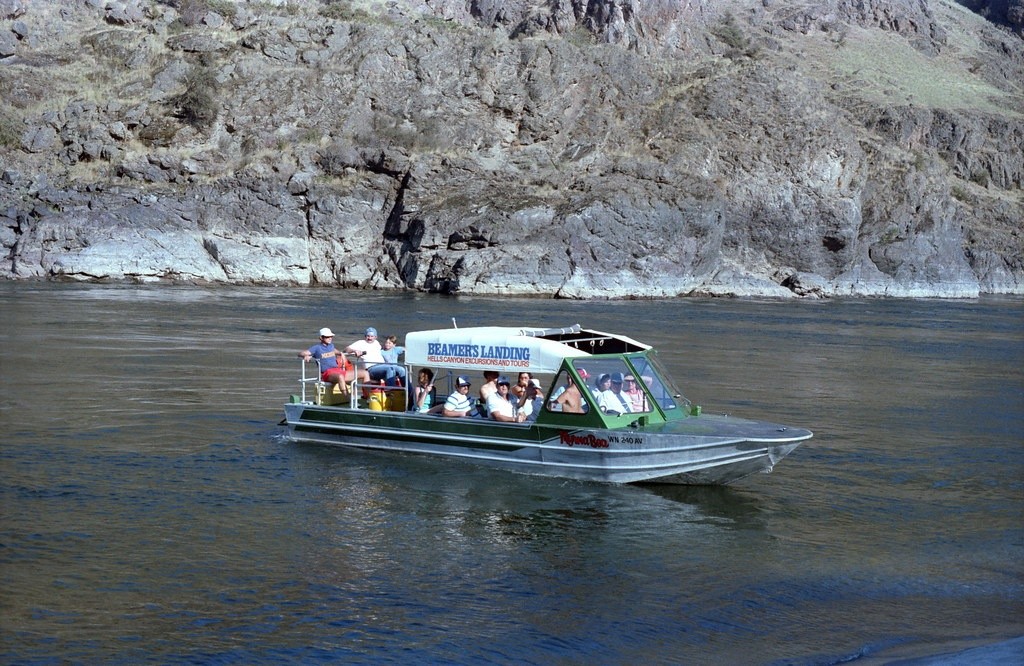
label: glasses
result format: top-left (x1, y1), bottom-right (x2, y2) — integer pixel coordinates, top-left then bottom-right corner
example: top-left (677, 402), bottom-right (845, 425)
top-left (324, 337), bottom-right (329, 338)
top-left (499, 383), bottom-right (509, 386)
top-left (613, 383), bottom-right (621, 384)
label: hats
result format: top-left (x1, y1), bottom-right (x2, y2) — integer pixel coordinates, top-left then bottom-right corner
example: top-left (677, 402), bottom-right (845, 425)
top-left (528, 379), bottom-right (542, 389)
top-left (610, 372), bottom-right (623, 383)
top-left (456, 375), bottom-right (472, 386)
top-left (319, 328), bottom-right (335, 337)
top-left (497, 376), bottom-right (510, 383)
top-left (596, 374), bottom-right (609, 385)
top-left (576, 368), bottom-right (591, 379)
top-left (624, 375), bottom-right (634, 381)
top-left (364, 327), bottom-right (377, 335)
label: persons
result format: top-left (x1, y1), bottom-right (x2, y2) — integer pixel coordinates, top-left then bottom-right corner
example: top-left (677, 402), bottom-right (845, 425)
top-left (442, 375), bottom-right (481, 418)
top-left (414, 368), bottom-right (436, 412)
top-left (298, 327), bottom-right (370, 407)
top-left (480, 371), bottom-right (500, 404)
top-left (344, 327), bottom-right (414, 395)
top-left (554, 367), bottom-right (652, 413)
top-left (485, 375), bottom-right (525, 422)
top-left (381, 335), bottom-right (405, 366)
top-left (511, 372), bottom-right (544, 398)
top-left (517, 380), bottom-right (544, 421)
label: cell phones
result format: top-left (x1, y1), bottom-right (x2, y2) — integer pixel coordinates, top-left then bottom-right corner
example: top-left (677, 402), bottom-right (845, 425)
top-left (362, 351), bottom-right (366, 355)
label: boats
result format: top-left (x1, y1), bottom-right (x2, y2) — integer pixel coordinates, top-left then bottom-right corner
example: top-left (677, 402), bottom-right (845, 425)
top-left (273, 315), bottom-right (814, 490)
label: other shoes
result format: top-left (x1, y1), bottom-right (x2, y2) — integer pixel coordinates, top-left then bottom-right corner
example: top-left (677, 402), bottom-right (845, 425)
top-left (349, 401), bottom-right (361, 407)
